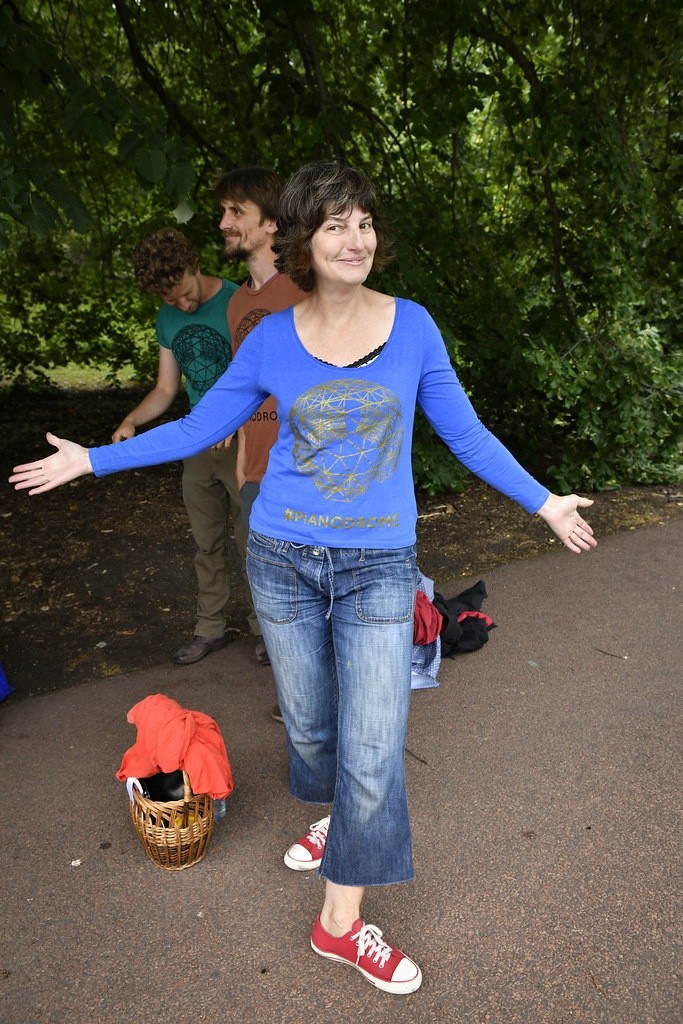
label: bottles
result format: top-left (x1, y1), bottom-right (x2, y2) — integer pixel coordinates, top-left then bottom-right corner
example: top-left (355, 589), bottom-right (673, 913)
top-left (214, 798), bottom-right (227, 820)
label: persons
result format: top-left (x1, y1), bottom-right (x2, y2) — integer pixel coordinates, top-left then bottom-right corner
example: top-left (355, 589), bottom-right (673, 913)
top-left (213, 164), bottom-right (312, 722)
top-left (111, 227), bottom-right (270, 663)
top-left (8, 167), bottom-right (597, 999)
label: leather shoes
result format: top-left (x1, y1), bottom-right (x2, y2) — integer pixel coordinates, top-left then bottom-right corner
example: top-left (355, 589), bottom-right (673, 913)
top-left (172, 637), bottom-right (227, 664)
top-left (257, 636), bottom-right (271, 665)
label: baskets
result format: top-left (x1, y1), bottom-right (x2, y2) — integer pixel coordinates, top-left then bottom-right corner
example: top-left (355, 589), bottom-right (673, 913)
top-left (126, 767), bottom-right (213, 870)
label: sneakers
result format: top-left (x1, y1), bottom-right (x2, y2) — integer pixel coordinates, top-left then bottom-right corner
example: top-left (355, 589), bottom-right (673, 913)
top-left (283, 814), bottom-right (331, 872)
top-left (311, 912), bottom-right (423, 995)
